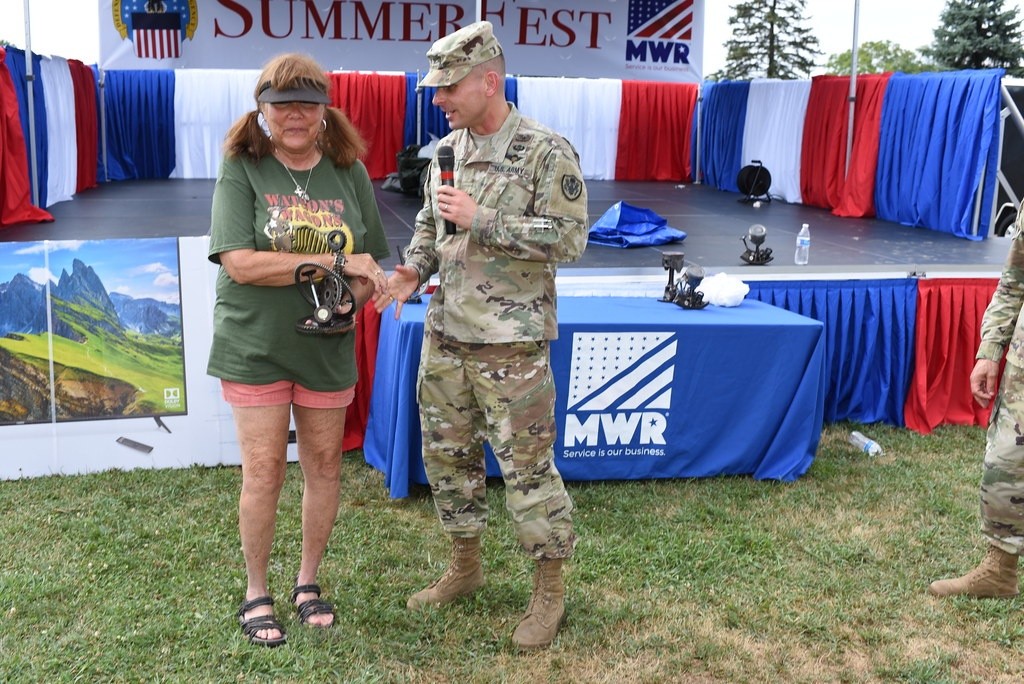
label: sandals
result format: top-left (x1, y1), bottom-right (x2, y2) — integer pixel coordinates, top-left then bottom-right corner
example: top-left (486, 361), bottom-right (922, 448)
top-left (234, 594), bottom-right (288, 648)
top-left (290, 581), bottom-right (337, 630)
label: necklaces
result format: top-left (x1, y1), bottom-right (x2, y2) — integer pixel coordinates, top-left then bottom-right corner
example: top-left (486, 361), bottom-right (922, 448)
top-left (272, 140), bottom-right (316, 202)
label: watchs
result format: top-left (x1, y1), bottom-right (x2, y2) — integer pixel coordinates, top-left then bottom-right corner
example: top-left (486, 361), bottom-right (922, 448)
top-left (340, 299), bottom-right (353, 305)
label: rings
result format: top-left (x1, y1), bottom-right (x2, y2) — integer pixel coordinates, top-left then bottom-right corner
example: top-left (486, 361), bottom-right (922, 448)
top-left (375, 271), bottom-right (381, 275)
top-left (389, 296), bottom-right (394, 302)
top-left (444, 204), bottom-right (448, 211)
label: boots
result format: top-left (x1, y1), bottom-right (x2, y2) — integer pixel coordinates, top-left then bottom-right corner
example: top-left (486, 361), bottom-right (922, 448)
top-left (512, 558), bottom-right (565, 649)
top-left (930, 545), bottom-right (1020, 599)
top-left (406, 535), bottom-right (484, 613)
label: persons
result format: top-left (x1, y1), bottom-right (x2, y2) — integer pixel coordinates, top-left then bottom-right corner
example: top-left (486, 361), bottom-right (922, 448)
top-left (371, 19), bottom-right (589, 654)
top-left (930, 196), bottom-right (1024, 598)
top-left (205, 56), bottom-right (390, 649)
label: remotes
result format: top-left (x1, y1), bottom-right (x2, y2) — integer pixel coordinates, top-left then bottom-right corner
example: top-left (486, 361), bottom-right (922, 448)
top-left (116, 437), bottom-right (154, 454)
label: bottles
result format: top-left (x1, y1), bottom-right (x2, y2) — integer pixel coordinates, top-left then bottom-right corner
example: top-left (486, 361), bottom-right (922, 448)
top-left (795, 223), bottom-right (812, 266)
top-left (849, 431), bottom-right (882, 456)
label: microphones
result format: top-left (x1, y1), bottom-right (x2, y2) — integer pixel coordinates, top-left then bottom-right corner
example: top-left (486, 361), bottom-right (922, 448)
top-left (438, 146), bottom-right (457, 235)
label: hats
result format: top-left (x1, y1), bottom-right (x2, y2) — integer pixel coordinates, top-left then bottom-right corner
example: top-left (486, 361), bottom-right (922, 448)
top-left (419, 21), bottom-right (502, 87)
top-left (256, 76), bottom-right (332, 104)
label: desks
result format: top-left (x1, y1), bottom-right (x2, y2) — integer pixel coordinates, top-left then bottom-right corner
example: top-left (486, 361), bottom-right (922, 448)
top-left (363, 292), bottom-right (828, 499)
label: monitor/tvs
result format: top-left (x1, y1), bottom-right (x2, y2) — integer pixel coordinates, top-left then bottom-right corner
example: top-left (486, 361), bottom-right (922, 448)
top-left (0, 241), bottom-right (188, 434)
